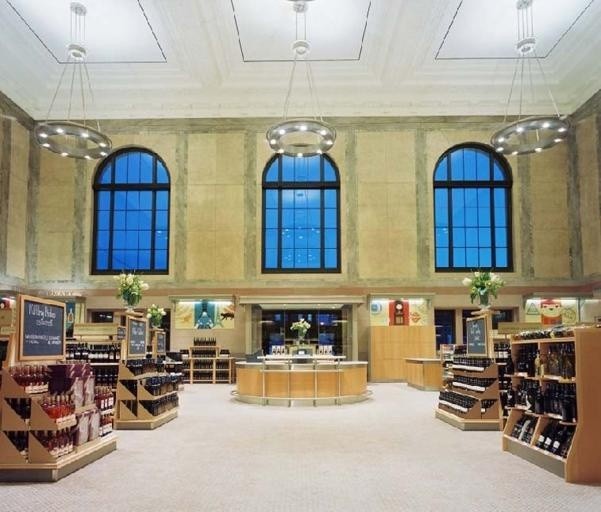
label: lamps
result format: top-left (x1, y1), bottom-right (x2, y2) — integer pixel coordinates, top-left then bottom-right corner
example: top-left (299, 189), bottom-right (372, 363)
top-left (32, 2), bottom-right (114, 160)
top-left (264, 1), bottom-right (338, 159)
top-left (488, 0), bottom-right (574, 154)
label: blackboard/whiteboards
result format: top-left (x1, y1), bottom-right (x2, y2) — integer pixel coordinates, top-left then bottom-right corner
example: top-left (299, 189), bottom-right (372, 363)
top-left (156, 332), bottom-right (166, 353)
top-left (19, 295), bottom-right (67, 361)
top-left (466, 315), bottom-right (488, 357)
top-left (127, 316), bottom-right (149, 357)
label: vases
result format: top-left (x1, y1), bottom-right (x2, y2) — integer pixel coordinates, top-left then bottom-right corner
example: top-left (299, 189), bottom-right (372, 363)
top-left (150, 321), bottom-right (163, 329)
top-left (479, 293), bottom-right (488, 307)
top-left (122, 296), bottom-right (137, 312)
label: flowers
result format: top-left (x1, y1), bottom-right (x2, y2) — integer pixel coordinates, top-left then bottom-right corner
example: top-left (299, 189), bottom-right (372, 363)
top-left (146, 304), bottom-right (167, 321)
top-left (462, 271), bottom-right (503, 304)
top-left (114, 270), bottom-right (149, 300)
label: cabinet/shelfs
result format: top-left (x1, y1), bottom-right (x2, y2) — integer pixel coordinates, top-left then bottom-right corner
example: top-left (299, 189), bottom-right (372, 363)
top-left (435, 359), bottom-right (501, 432)
top-left (0, 291), bottom-right (183, 481)
top-left (501, 325), bottom-right (601, 484)
top-left (183, 345), bottom-right (236, 384)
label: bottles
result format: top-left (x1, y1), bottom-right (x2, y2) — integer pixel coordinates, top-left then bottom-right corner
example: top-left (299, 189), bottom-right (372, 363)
top-left (3, 336), bottom-right (232, 458)
top-left (438, 325), bottom-right (577, 457)
